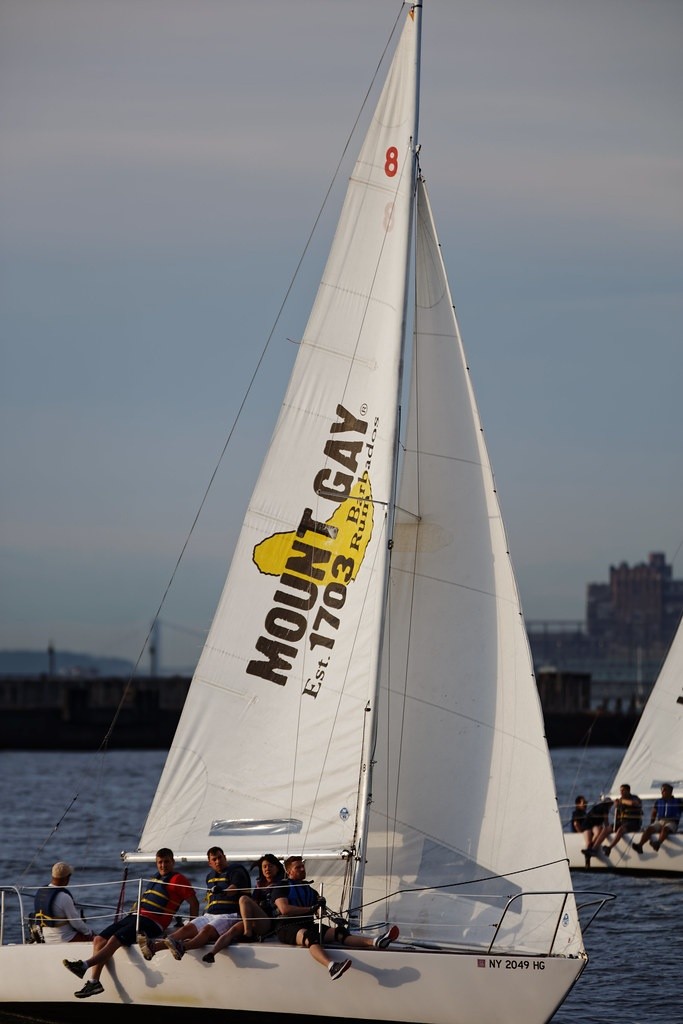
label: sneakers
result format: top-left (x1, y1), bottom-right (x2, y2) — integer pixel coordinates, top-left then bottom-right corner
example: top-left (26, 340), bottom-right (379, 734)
top-left (202, 952), bottom-right (215, 963)
top-left (329, 959), bottom-right (352, 980)
top-left (63, 959), bottom-right (87, 979)
top-left (74, 980), bottom-right (104, 998)
top-left (137, 931), bottom-right (156, 961)
top-left (164, 937), bottom-right (187, 960)
top-left (375, 925), bottom-right (399, 949)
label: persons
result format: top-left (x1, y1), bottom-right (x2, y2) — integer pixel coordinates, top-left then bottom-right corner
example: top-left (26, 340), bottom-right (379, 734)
top-left (581, 784), bottom-right (642, 858)
top-left (270, 855), bottom-right (399, 981)
top-left (201, 854), bottom-right (285, 963)
top-left (34, 862), bottom-right (97, 943)
top-left (63, 848), bottom-right (199, 999)
top-left (571, 796), bottom-right (592, 849)
top-left (138, 846), bottom-right (251, 960)
top-left (632, 784), bottom-right (681, 854)
top-left (587, 798), bottom-right (613, 851)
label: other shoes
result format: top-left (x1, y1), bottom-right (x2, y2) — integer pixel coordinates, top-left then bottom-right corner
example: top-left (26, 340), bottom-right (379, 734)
top-left (602, 845), bottom-right (611, 857)
top-left (632, 843), bottom-right (644, 854)
top-left (581, 849), bottom-right (599, 856)
top-left (649, 840), bottom-right (661, 852)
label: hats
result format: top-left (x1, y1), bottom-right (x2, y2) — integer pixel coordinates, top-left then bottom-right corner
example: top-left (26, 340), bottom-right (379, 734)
top-left (52, 863), bottom-right (75, 878)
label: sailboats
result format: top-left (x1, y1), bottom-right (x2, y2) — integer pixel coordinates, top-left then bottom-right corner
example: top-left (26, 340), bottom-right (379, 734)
top-left (555, 613), bottom-right (683, 878)
top-left (0, 0), bottom-right (624, 1024)
